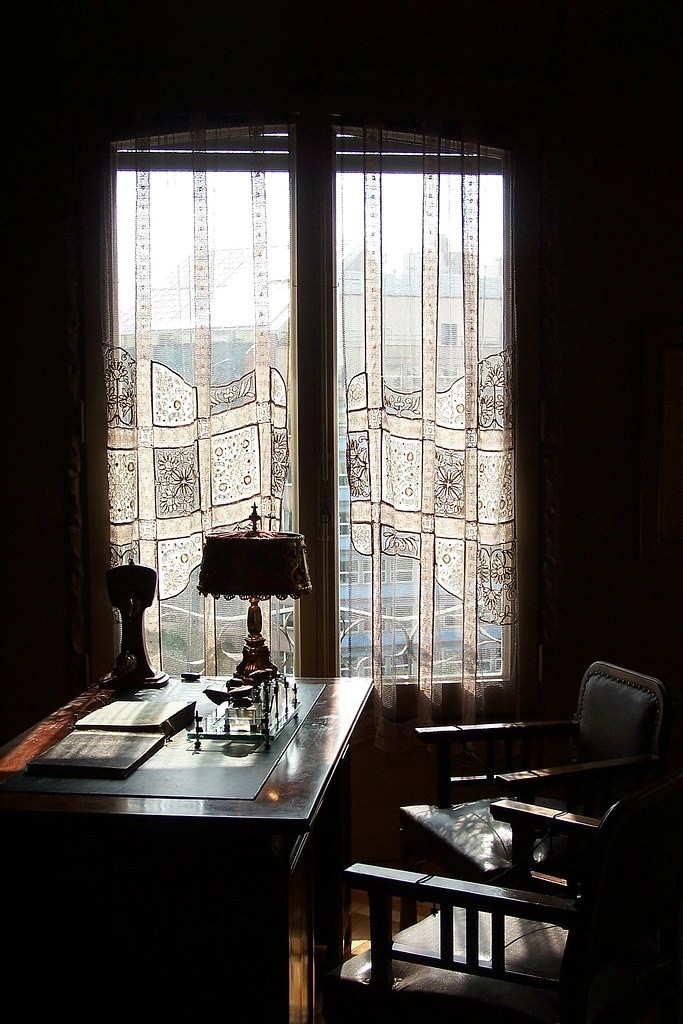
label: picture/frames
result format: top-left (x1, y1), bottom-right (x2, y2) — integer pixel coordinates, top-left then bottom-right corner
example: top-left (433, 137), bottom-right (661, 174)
top-left (637, 323), bottom-right (683, 566)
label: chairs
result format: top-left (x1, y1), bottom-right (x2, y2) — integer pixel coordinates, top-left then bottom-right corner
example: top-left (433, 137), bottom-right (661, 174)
top-left (324, 662), bottom-right (676, 1024)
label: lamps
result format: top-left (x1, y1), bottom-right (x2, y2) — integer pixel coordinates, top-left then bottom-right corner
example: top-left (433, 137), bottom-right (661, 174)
top-left (196, 502), bottom-right (312, 686)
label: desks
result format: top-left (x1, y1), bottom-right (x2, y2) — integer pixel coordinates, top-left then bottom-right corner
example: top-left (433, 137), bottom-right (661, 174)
top-left (0, 677), bottom-right (376, 1024)
